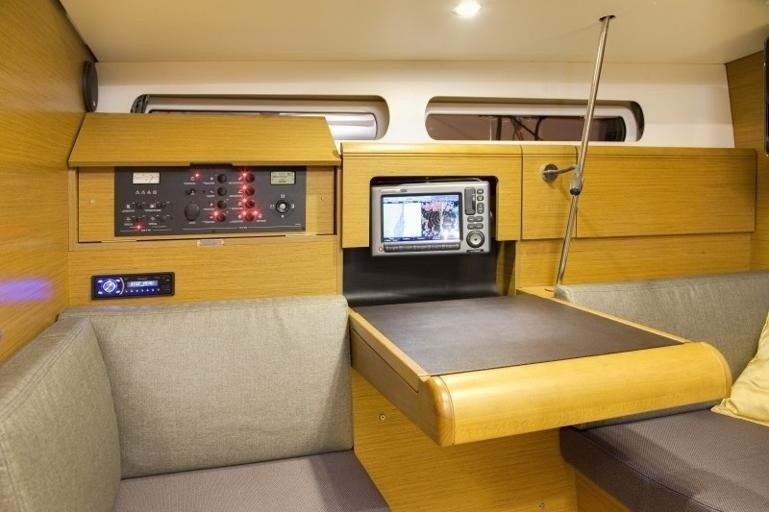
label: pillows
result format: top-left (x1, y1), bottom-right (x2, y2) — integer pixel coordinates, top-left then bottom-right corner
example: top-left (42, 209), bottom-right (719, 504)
top-left (711, 309), bottom-right (768, 427)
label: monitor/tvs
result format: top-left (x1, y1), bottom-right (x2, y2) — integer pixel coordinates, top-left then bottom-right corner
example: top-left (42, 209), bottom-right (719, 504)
top-left (369, 178), bottom-right (492, 259)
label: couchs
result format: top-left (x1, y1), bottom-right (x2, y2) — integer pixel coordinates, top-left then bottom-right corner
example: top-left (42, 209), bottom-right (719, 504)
top-left (554, 268), bottom-right (769, 512)
top-left (1, 293), bottom-right (392, 510)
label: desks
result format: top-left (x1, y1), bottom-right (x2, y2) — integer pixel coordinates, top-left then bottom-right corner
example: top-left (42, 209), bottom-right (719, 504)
top-left (346, 292), bottom-right (731, 450)
